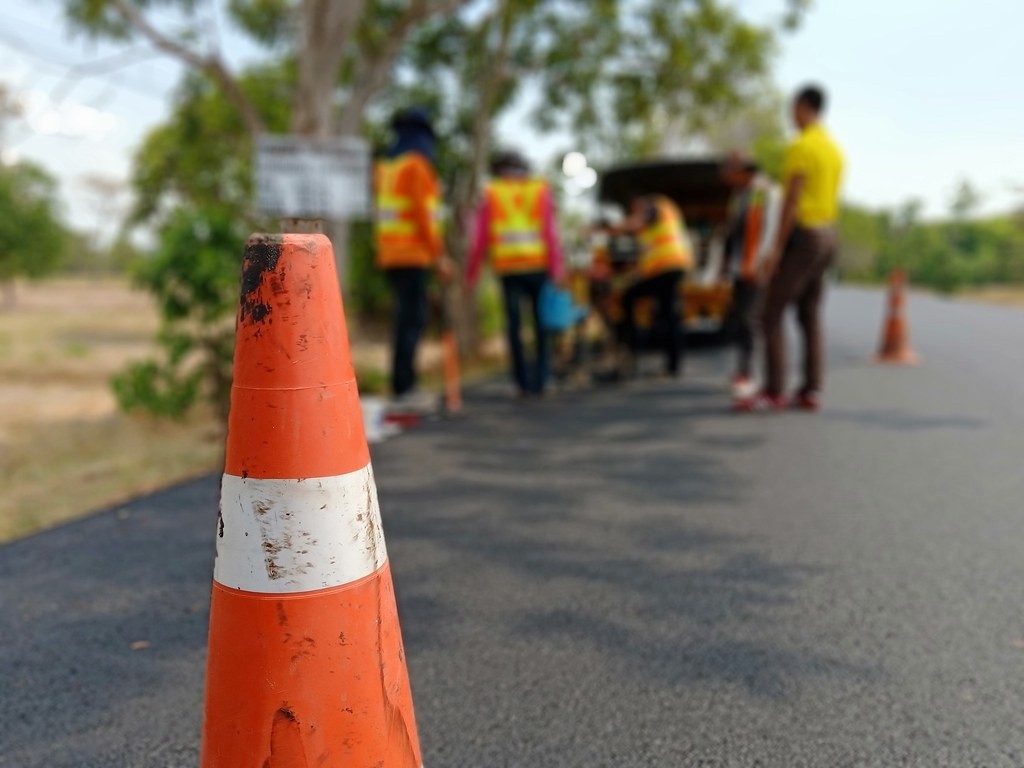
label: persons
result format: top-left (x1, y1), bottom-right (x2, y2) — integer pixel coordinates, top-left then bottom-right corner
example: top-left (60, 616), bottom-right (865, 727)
top-left (465, 150), bottom-right (568, 399)
top-left (368, 104), bottom-right (462, 415)
top-left (717, 85), bottom-right (841, 412)
top-left (589, 186), bottom-right (688, 384)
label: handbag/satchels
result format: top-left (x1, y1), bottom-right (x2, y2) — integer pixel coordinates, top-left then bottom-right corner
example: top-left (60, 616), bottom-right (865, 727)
top-left (540, 282), bottom-right (583, 329)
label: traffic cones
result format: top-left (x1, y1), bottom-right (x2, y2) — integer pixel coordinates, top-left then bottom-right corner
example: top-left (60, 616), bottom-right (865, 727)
top-left (865, 267), bottom-right (919, 368)
top-left (199, 229), bottom-right (427, 765)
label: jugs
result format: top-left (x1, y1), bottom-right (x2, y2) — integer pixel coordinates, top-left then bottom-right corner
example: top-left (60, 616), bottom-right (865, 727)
top-left (538, 288), bottom-right (588, 329)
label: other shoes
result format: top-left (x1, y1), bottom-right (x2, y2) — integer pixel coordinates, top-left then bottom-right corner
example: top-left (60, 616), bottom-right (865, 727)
top-left (392, 389), bottom-right (435, 411)
top-left (797, 390), bottom-right (817, 409)
top-left (734, 391), bottom-right (787, 411)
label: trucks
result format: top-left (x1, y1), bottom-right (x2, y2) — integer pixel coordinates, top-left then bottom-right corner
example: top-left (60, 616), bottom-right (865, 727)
top-left (585, 152), bottom-right (752, 348)
